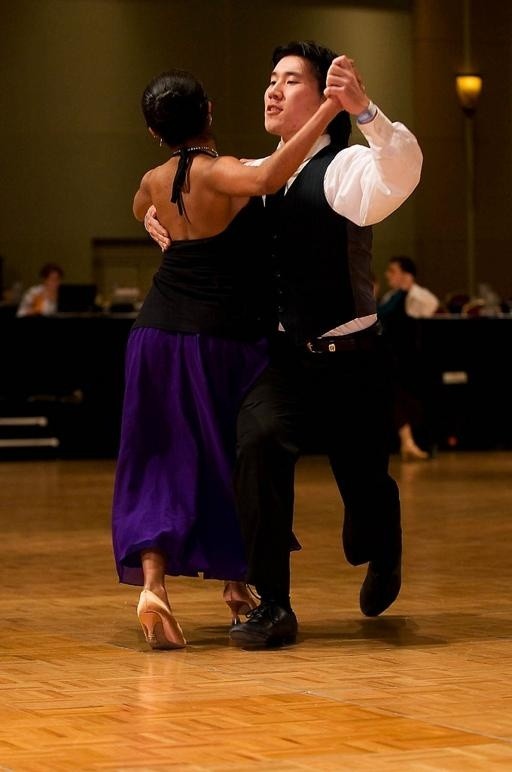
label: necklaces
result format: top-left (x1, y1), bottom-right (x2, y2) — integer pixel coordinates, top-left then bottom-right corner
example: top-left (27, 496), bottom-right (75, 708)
top-left (172, 146), bottom-right (219, 157)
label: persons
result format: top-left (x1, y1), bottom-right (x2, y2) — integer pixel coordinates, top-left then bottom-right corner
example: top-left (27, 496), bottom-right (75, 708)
top-left (140, 40), bottom-right (423, 649)
top-left (381, 253), bottom-right (440, 317)
top-left (16, 264), bottom-right (65, 318)
top-left (385, 389), bottom-right (431, 463)
top-left (104, 58), bottom-right (371, 653)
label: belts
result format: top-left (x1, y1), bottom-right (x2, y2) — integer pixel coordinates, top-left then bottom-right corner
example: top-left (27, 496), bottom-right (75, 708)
top-left (272, 319), bottom-right (383, 360)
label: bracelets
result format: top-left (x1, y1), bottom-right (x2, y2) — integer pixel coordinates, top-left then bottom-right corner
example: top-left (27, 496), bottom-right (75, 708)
top-left (357, 99), bottom-right (377, 123)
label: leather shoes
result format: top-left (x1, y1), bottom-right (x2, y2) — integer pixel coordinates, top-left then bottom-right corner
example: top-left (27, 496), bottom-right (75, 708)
top-left (360, 545), bottom-right (402, 617)
top-left (229, 603), bottom-right (298, 649)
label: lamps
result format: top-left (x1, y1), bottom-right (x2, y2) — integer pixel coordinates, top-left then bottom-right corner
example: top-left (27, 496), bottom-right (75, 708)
top-left (455, 74), bottom-right (483, 117)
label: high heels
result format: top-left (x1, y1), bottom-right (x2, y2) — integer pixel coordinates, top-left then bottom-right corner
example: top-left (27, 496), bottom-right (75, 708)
top-left (221, 580), bottom-right (260, 625)
top-left (136, 590), bottom-right (188, 651)
top-left (400, 441), bottom-right (428, 462)
